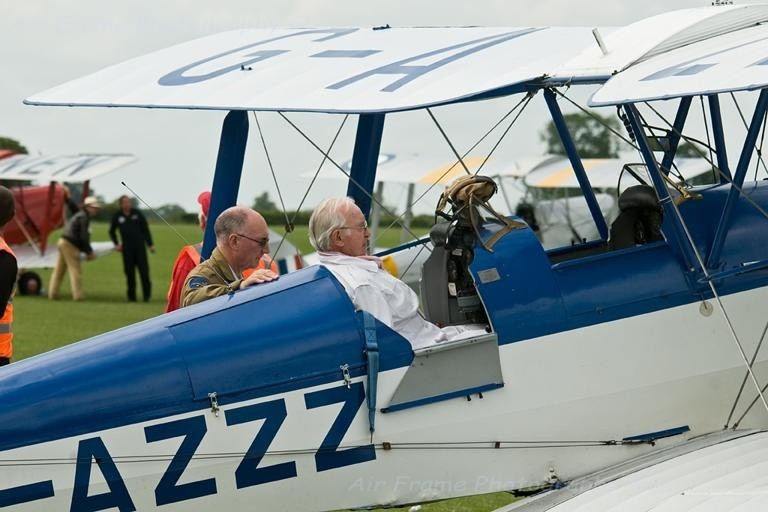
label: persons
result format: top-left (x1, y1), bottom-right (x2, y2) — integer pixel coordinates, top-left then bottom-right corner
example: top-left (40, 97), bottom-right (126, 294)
top-left (47, 196), bottom-right (102, 300)
top-left (109, 196), bottom-right (157, 303)
top-left (166, 191), bottom-right (280, 312)
top-left (0, 184), bottom-right (19, 366)
top-left (307, 196), bottom-right (491, 352)
top-left (179, 205), bottom-right (279, 308)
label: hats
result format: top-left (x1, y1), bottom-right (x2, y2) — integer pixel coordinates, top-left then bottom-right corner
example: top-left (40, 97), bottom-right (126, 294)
top-left (85, 196), bottom-right (102, 209)
top-left (0, 185), bottom-right (15, 227)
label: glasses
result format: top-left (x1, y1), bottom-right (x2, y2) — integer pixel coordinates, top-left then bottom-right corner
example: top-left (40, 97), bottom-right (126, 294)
top-left (234, 233), bottom-right (270, 248)
top-left (329, 221), bottom-right (368, 238)
